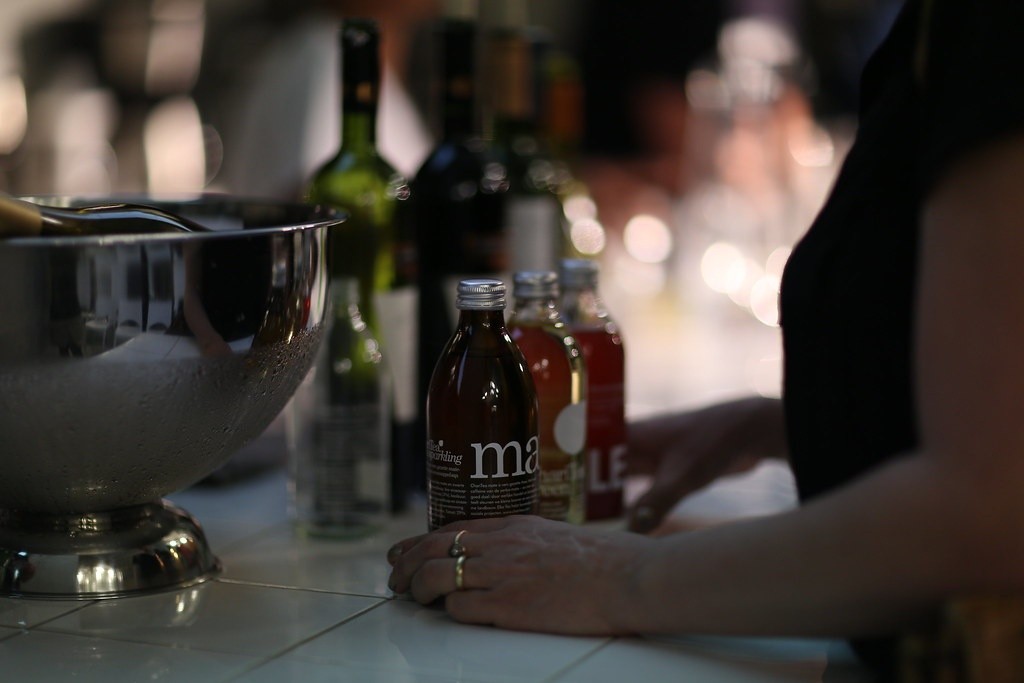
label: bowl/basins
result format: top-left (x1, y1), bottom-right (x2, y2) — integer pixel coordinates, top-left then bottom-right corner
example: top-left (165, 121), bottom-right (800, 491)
top-left (0, 197), bottom-right (346, 598)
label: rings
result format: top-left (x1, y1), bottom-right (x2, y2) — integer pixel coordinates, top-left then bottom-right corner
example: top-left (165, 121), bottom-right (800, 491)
top-left (448, 529), bottom-right (467, 555)
top-left (454, 558), bottom-right (465, 590)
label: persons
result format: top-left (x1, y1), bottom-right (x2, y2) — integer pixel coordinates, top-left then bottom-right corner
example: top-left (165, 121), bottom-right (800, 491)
top-left (389, 0), bottom-right (1023, 683)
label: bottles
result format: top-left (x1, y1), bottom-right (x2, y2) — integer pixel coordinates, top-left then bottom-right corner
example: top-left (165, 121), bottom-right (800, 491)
top-left (300, 18), bottom-right (403, 540)
top-left (488, 31), bottom-right (566, 313)
top-left (508, 272), bottom-right (576, 520)
top-left (555, 259), bottom-right (624, 523)
top-left (425, 278), bottom-right (539, 533)
top-left (406, 20), bottom-right (509, 371)
